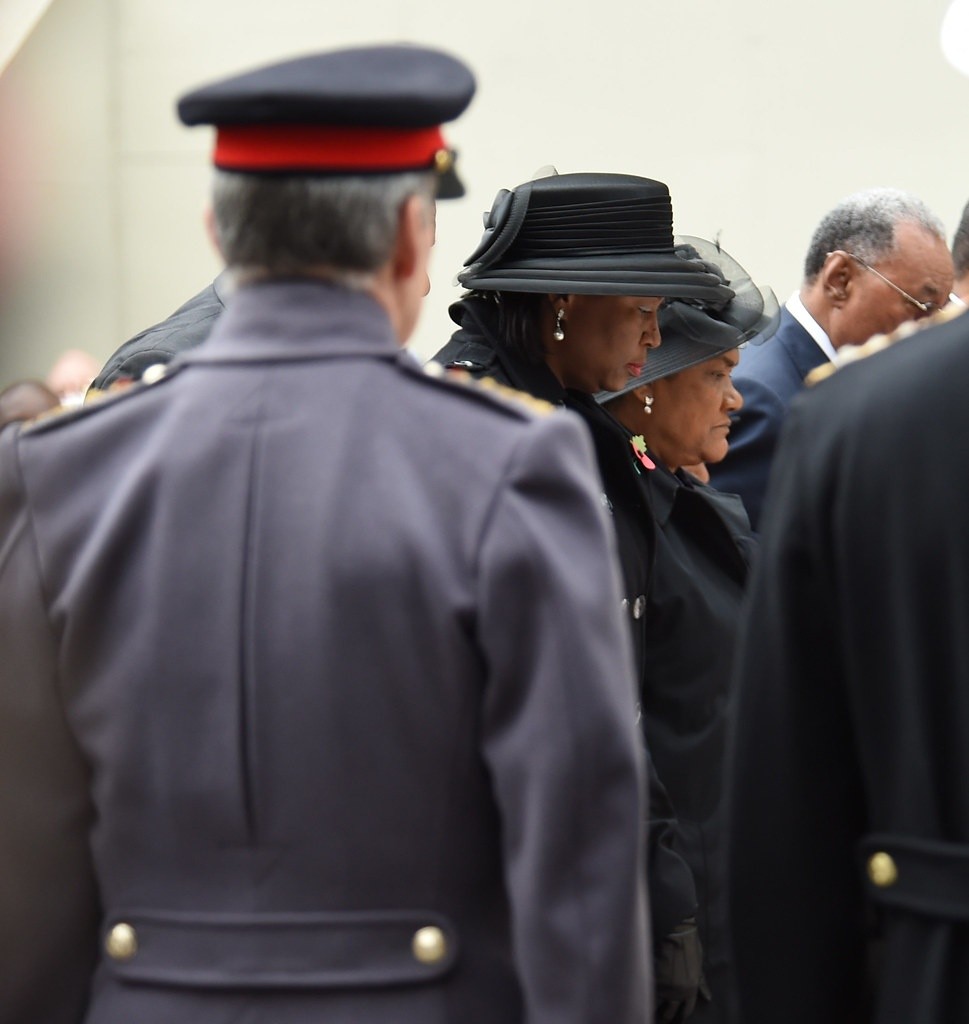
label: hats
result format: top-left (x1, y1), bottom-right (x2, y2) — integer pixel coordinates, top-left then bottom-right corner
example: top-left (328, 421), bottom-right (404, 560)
top-left (458, 173), bottom-right (736, 301)
top-left (592, 236), bottom-right (782, 404)
top-left (180, 47), bottom-right (474, 199)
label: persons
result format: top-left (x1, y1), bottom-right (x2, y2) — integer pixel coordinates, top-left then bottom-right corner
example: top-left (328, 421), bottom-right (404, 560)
top-left (0, 42), bottom-right (968, 1024)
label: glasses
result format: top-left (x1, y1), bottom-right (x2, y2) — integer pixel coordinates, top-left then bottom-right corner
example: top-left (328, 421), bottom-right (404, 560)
top-left (826, 252), bottom-right (938, 322)
